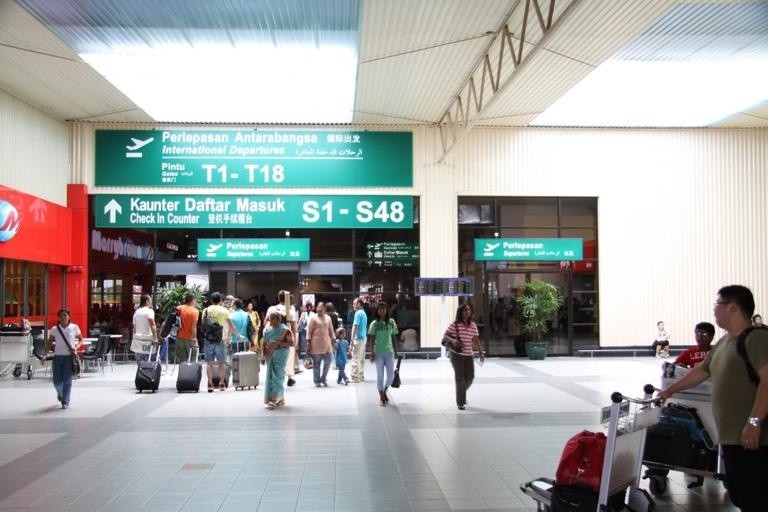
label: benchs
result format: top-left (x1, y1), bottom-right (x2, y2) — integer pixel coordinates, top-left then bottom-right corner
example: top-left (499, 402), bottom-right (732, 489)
top-left (578, 345), bottom-right (698, 356)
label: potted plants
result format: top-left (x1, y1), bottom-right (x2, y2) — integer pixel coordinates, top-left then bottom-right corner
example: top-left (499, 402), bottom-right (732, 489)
top-left (516, 280), bottom-right (564, 360)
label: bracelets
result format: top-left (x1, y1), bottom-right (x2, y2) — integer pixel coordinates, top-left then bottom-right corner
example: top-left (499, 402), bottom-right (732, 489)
top-left (747, 416), bottom-right (762, 427)
top-left (76, 345), bottom-right (79, 350)
top-left (479, 351), bottom-right (482, 354)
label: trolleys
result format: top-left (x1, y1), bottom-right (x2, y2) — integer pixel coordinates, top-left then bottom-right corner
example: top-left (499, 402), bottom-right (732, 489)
top-left (518, 386), bottom-right (665, 511)
top-left (648, 374), bottom-right (729, 495)
top-left (0, 326), bottom-right (34, 380)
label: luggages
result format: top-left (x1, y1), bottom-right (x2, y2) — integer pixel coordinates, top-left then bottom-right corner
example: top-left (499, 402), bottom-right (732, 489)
top-left (212, 348), bottom-right (231, 387)
top-left (176, 346), bottom-right (202, 392)
top-left (135, 342), bottom-right (161, 392)
top-left (232, 338), bottom-right (258, 391)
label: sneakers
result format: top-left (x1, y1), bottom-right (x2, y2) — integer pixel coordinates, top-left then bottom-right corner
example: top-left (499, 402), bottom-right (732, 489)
top-left (294, 370), bottom-right (302, 374)
top-left (268, 399), bottom-right (284, 409)
top-left (58, 394), bottom-right (69, 409)
top-left (458, 405), bottom-right (466, 410)
top-left (208, 382), bottom-right (225, 392)
top-left (379, 396), bottom-right (389, 405)
top-left (315, 379), bottom-right (360, 386)
top-left (287, 379), bottom-right (296, 386)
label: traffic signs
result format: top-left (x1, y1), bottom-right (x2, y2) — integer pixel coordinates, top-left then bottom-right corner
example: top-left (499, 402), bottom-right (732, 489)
top-left (95, 195), bottom-right (414, 228)
top-left (94, 128), bottom-right (412, 189)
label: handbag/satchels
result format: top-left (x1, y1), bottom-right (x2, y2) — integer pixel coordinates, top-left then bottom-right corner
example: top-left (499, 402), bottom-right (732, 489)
top-left (303, 358), bottom-right (314, 369)
top-left (160, 312), bottom-right (182, 338)
top-left (201, 318), bottom-right (223, 343)
top-left (442, 334), bottom-right (464, 353)
top-left (643, 402), bottom-right (716, 472)
top-left (554, 428), bottom-right (607, 502)
top-left (247, 321), bottom-right (255, 339)
top-left (71, 357), bottom-right (81, 376)
top-left (391, 369), bottom-right (401, 388)
top-left (297, 318), bottom-right (307, 332)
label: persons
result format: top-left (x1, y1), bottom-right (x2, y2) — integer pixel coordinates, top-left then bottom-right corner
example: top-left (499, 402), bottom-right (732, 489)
top-left (654, 286), bottom-right (768, 512)
top-left (132, 294), bottom-right (159, 366)
top-left (652, 321), bottom-right (670, 358)
top-left (752, 314), bottom-right (767, 331)
top-left (262, 312), bottom-right (295, 409)
top-left (367, 302), bottom-right (401, 403)
top-left (174, 290), bottom-right (420, 392)
top-left (444, 303), bottom-right (484, 410)
top-left (492, 297), bottom-right (506, 337)
top-left (674, 321), bottom-right (715, 368)
top-left (42, 309), bottom-right (83, 409)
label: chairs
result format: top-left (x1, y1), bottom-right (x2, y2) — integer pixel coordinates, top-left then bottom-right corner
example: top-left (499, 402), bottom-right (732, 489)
top-left (33, 327), bottom-right (129, 377)
